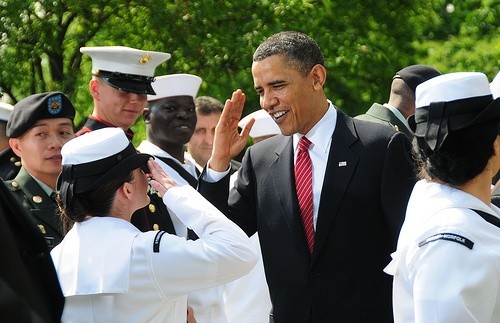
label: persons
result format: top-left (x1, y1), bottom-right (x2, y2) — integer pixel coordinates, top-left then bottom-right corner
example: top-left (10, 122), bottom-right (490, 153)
top-left (0, 45), bottom-right (500, 323)
top-left (193, 31), bottom-right (413, 323)
top-left (351, 65), bottom-right (443, 139)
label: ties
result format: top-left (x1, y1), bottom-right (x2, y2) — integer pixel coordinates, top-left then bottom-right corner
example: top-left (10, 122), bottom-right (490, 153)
top-left (52, 191), bottom-right (57, 204)
top-left (295, 136), bottom-right (315, 255)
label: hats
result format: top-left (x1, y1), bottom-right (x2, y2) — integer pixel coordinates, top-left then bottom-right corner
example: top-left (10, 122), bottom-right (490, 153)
top-left (81, 45), bottom-right (172, 95)
top-left (6, 92), bottom-right (75, 138)
top-left (0, 102), bottom-right (14, 122)
top-left (55, 124), bottom-right (154, 196)
top-left (398, 66), bottom-right (440, 95)
top-left (406, 71), bottom-right (500, 151)
top-left (148, 74), bottom-right (203, 101)
